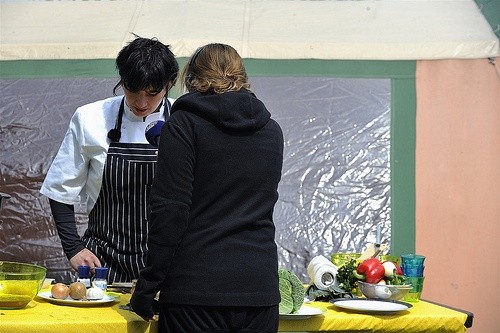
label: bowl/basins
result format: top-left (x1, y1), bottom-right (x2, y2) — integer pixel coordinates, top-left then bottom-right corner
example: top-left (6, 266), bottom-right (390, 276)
top-left (0, 261), bottom-right (47, 310)
top-left (357, 280), bottom-right (414, 300)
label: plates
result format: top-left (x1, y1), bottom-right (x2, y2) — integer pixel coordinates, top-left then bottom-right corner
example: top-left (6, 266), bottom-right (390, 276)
top-left (36, 291), bottom-right (117, 306)
top-left (279, 305), bottom-right (323, 320)
top-left (330, 297), bottom-right (413, 314)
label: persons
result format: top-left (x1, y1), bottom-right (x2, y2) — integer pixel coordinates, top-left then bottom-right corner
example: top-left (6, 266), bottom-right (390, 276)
top-left (39, 37), bottom-right (180, 289)
top-left (129, 42), bottom-right (284, 333)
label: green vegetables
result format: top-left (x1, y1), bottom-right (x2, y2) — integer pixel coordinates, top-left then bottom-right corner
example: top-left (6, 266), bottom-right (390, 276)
top-left (307, 259), bottom-right (359, 301)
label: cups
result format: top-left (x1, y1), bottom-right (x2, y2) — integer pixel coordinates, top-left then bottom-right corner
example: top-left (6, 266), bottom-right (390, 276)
top-left (401, 254), bottom-right (425, 303)
top-left (380, 255), bottom-right (400, 266)
top-left (332, 253), bottom-right (361, 295)
top-left (43, 279), bottom-right (55, 288)
top-left (132, 280), bottom-right (137, 295)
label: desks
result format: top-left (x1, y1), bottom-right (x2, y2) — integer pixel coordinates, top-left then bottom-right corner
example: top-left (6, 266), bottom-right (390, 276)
top-left (0, 284), bottom-right (474, 333)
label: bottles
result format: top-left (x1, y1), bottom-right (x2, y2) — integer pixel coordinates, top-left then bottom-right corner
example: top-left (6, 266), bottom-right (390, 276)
top-left (92, 267), bottom-right (108, 295)
top-left (78, 265), bottom-right (90, 286)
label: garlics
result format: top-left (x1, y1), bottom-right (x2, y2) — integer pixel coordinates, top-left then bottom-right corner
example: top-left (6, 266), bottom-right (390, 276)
top-left (86, 287), bottom-right (103, 300)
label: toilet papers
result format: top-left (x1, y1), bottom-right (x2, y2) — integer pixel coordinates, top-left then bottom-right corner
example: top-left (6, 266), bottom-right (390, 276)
top-left (306, 254), bottom-right (341, 291)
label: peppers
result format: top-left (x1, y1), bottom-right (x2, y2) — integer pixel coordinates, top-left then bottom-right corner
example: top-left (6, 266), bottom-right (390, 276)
top-left (352, 259), bottom-right (406, 285)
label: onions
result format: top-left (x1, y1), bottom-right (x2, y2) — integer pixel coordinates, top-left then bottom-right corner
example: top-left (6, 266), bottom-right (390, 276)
top-left (52, 281), bottom-right (87, 299)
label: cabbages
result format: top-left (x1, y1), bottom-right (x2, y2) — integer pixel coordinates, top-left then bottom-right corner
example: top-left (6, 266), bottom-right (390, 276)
top-left (278, 268), bottom-right (305, 314)
